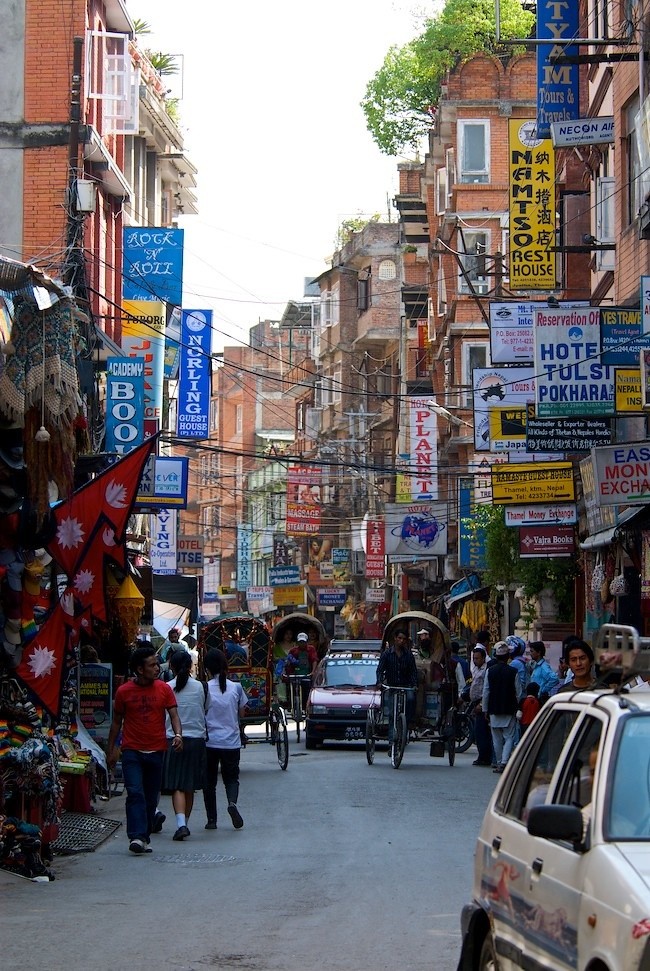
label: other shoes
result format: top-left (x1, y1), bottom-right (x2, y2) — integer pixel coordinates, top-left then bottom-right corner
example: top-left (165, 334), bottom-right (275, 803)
top-left (205, 822), bottom-right (216, 829)
top-left (144, 841), bottom-right (152, 852)
top-left (153, 811), bottom-right (166, 833)
top-left (129, 839), bottom-right (145, 853)
top-left (173, 826), bottom-right (190, 840)
top-left (473, 759), bottom-right (491, 765)
top-left (500, 763), bottom-right (506, 774)
top-left (493, 767), bottom-right (500, 773)
top-left (227, 805), bottom-right (244, 828)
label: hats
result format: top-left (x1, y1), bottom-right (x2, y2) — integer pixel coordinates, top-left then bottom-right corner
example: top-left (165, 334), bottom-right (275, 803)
top-left (496, 643), bottom-right (508, 656)
top-left (416, 629), bottom-right (429, 635)
top-left (297, 633), bottom-right (308, 642)
top-left (494, 641), bottom-right (514, 650)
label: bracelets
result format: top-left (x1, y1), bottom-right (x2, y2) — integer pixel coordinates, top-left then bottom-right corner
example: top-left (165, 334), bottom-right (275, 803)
top-left (175, 734), bottom-right (183, 741)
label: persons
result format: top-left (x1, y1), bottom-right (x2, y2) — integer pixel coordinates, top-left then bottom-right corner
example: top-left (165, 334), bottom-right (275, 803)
top-left (280, 633), bottom-right (318, 712)
top-left (581, 738), bottom-right (637, 844)
top-left (376, 629), bottom-right (419, 757)
top-left (161, 629), bottom-right (185, 663)
top-left (105, 647), bottom-right (183, 853)
top-left (163, 650), bottom-right (205, 841)
top-left (451, 636), bottom-right (611, 773)
top-left (200, 647), bottom-right (248, 830)
top-left (310, 539), bottom-right (333, 563)
top-left (223, 628), bottom-right (247, 666)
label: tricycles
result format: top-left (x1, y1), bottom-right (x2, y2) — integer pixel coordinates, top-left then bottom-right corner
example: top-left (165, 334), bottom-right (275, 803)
top-left (366, 611), bottom-right (458, 769)
top-left (270, 613), bottom-right (329, 743)
top-left (192, 612), bottom-right (288, 770)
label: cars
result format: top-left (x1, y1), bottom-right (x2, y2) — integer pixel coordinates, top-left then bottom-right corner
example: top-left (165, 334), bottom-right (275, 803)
top-left (455, 684), bottom-right (650, 971)
top-left (300, 638), bottom-right (382, 750)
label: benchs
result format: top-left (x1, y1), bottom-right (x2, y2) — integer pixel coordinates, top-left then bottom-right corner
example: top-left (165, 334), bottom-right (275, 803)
top-left (521, 776), bottom-right (591, 824)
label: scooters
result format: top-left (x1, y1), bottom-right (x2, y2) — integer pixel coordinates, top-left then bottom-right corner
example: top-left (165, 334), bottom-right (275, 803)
top-left (443, 678), bottom-right (475, 754)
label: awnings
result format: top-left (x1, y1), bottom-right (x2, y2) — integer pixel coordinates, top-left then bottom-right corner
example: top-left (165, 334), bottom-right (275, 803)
top-left (580, 505), bottom-right (646, 549)
top-left (444, 572), bottom-right (504, 609)
